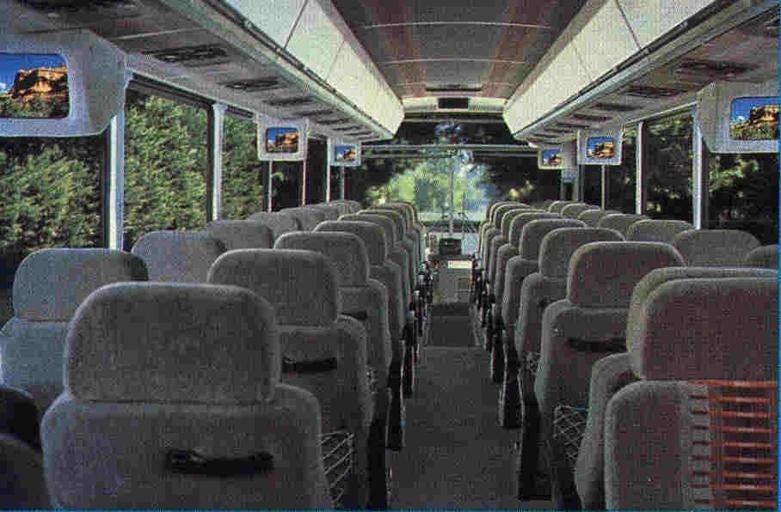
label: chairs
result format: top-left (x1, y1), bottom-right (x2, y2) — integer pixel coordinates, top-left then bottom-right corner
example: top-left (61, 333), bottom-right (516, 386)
top-left (469, 200), bottom-right (781, 511)
top-left (0, 200), bottom-right (437, 512)
